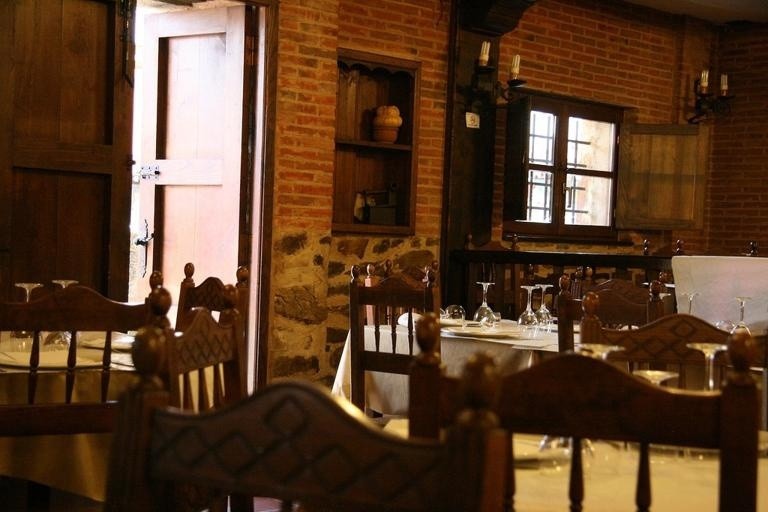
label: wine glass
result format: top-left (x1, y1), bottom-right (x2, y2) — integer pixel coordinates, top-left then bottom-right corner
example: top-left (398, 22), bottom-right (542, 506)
top-left (472, 282), bottom-right (496, 330)
top-left (10, 279), bottom-right (80, 359)
top-left (730, 296), bottom-right (752, 342)
top-left (534, 340), bottom-right (727, 480)
top-left (681, 291), bottom-right (699, 317)
top-left (438, 305), bottom-right (465, 322)
top-left (518, 280), bottom-right (551, 332)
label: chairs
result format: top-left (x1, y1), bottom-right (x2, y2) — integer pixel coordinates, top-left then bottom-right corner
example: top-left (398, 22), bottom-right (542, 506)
top-left (0, 233), bottom-right (758, 512)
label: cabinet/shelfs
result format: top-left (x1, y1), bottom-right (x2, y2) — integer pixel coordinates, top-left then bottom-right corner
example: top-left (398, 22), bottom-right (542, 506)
top-left (332, 46), bottom-right (422, 237)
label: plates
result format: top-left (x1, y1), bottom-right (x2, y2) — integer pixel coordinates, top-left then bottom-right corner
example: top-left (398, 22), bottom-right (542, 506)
top-left (542, 323), bottom-right (580, 335)
top-left (445, 326), bottom-right (523, 340)
top-left (434, 320), bottom-right (481, 327)
